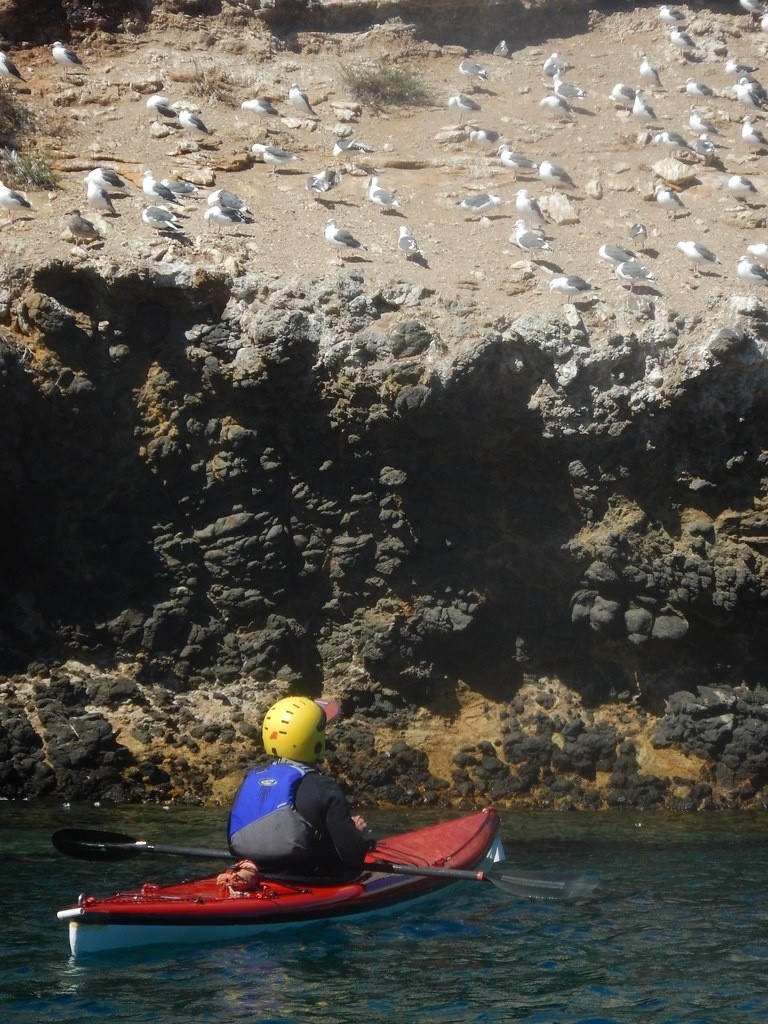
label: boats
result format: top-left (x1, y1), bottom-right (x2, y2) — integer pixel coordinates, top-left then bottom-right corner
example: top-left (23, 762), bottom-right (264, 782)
top-left (57, 805), bottom-right (508, 957)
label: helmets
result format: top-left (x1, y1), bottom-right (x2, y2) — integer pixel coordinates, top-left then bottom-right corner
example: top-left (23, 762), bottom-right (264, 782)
top-left (261, 695), bottom-right (326, 765)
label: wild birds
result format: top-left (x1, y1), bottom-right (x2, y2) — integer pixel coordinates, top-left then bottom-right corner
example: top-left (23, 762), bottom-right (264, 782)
top-left (1, 0), bottom-right (766, 304)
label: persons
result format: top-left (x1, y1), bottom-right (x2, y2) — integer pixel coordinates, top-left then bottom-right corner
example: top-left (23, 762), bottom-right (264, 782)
top-left (227, 696), bottom-right (367, 881)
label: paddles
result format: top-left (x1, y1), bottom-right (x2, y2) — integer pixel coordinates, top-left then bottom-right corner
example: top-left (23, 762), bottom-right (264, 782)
top-left (50, 827), bottom-right (602, 905)
